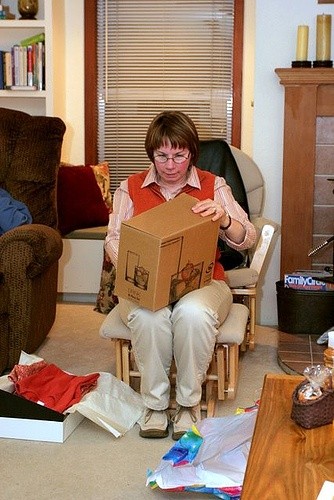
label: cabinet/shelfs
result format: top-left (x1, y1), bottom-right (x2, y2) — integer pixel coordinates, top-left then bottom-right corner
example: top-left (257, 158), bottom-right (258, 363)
top-left (0, 0), bottom-right (51, 117)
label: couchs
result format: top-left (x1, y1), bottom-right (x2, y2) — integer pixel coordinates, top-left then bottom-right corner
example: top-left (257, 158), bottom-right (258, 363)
top-left (0, 107), bottom-right (66, 376)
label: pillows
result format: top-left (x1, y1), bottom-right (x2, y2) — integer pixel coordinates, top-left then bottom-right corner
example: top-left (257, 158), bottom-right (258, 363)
top-left (58, 159), bottom-right (112, 213)
top-left (57, 166), bottom-right (109, 234)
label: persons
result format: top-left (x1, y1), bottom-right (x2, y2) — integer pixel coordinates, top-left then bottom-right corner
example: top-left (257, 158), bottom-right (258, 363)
top-left (105, 111), bottom-right (256, 439)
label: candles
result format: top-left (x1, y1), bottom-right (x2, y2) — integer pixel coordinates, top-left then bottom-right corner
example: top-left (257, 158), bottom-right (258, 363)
top-left (296, 25), bottom-right (308, 61)
top-left (317, 13), bottom-right (332, 61)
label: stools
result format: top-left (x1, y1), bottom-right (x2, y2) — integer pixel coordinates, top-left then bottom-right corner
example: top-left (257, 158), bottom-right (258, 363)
top-left (97, 303), bottom-right (251, 420)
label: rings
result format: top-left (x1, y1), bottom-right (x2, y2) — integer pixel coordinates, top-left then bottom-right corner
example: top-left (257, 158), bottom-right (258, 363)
top-left (212, 207), bottom-right (217, 213)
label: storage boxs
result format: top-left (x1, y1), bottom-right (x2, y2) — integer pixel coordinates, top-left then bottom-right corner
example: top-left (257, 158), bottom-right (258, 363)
top-left (114, 193), bottom-right (220, 309)
top-left (0, 409), bottom-right (84, 443)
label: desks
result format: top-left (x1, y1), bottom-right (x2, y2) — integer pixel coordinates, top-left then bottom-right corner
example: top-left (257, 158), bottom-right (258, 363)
top-left (242, 373), bottom-right (334, 500)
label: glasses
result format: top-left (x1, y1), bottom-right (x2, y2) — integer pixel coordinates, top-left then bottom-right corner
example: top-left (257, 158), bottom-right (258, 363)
top-left (151, 151), bottom-right (191, 164)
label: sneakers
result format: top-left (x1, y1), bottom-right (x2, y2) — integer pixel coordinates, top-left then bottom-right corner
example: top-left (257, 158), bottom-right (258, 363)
top-left (171, 404), bottom-right (201, 440)
top-left (139, 408), bottom-right (171, 437)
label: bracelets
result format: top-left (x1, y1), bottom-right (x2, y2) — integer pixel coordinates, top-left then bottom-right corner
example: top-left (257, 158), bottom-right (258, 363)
top-left (220, 213), bottom-right (231, 230)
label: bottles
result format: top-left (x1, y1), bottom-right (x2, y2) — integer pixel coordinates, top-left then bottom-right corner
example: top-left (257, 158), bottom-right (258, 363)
top-left (18, 0), bottom-right (38, 20)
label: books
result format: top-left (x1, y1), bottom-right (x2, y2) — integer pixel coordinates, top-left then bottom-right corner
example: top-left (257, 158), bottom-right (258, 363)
top-left (0, 32), bottom-right (45, 91)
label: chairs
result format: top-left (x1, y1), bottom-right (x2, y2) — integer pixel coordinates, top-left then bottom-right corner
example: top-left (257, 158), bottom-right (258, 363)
top-left (222, 145), bottom-right (279, 352)
